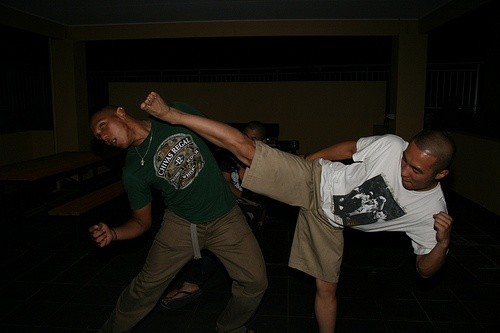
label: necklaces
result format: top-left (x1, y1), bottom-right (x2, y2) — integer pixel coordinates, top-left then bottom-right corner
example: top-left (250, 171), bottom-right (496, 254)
top-left (133, 123), bottom-right (158, 167)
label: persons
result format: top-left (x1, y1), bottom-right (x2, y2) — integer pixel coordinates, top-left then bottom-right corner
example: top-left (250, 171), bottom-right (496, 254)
top-left (139, 65), bottom-right (454, 333)
top-left (157, 119), bottom-right (270, 310)
top-left (215, 121), bottom-right (268, 229)
top-left (84, 92), bottom-right (270, 332)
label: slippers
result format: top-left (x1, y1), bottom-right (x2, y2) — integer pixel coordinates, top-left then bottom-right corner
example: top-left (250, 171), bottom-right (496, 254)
top-left (160, 283), bottom-right (202, 310)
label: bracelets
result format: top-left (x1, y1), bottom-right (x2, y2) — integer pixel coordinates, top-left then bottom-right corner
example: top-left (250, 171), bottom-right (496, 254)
top-left (303, 152), bottom-right (311, 159)
top-left (111, 228), bottom-right (118, 241)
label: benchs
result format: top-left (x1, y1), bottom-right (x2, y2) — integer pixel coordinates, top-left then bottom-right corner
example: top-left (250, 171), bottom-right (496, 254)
top-left (47, 179), bottom-right (129, 236)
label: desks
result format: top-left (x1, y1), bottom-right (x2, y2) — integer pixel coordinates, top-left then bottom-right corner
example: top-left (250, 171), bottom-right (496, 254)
top-left (1, 148), bottom-right (116, 209)
